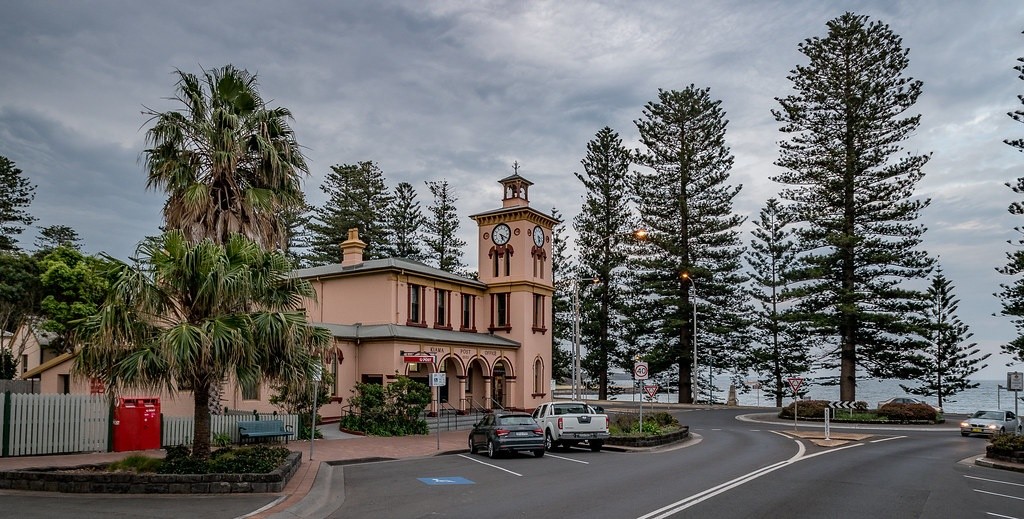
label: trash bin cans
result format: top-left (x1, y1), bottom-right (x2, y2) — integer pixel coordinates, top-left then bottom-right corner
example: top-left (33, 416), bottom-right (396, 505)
top-left (112, 396), bottom-right (161, 452)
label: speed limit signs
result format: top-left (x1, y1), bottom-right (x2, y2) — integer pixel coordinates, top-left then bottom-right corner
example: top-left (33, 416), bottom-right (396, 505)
top-left (634, 362), bottom-right (648, 380)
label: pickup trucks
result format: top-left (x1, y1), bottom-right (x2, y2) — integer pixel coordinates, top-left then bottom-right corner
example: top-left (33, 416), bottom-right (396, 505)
top-left (533, 401), bottom-right (611, 451)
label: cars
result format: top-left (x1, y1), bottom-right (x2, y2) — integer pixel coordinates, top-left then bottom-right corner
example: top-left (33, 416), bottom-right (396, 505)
top-left (960, 411), bottom-right (1022, 437)
top-left (468, 410), bottom-right (546, 458)
top-left (877, 397), bottom-right (943, 413)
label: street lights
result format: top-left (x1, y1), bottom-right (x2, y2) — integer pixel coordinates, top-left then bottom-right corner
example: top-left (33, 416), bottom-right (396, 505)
top-left (683, 273), bottom-right (699, 404)
top-left (575, 231), bottom-right (645, 401)
top-left (570, 278), bottom-right (600, 401)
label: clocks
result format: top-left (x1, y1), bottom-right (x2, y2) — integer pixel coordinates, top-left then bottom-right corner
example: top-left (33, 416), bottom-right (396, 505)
top-left (533, 225), bottom-right (544, 248)
top-left (492, 223), bottom-right (511, 246)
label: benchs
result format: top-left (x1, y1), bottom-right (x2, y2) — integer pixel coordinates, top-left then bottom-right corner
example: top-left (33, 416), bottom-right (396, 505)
top-left (236, 420), bottom-right (294, 447)
